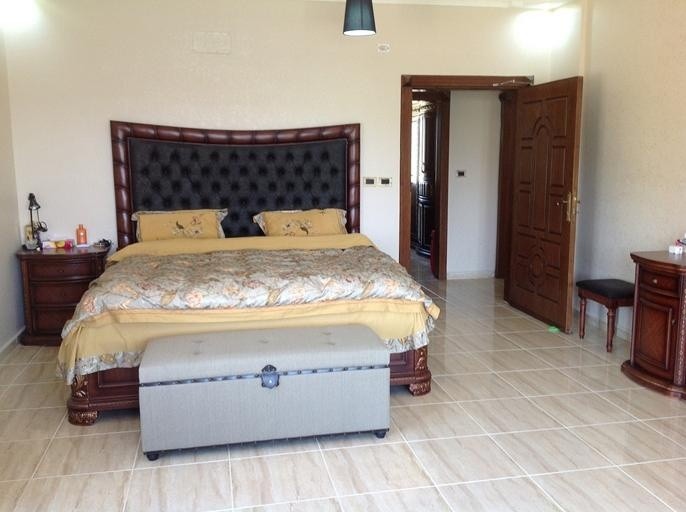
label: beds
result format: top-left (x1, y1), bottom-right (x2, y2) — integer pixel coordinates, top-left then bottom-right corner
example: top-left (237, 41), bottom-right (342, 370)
top-left (48, 116), bottom-right (445, 427)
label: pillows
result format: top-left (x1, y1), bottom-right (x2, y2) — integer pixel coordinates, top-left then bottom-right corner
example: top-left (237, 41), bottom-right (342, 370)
top-left (128, 206), bottom-right (229, 243)
top-left (252, 206), bottom-right (350, 241)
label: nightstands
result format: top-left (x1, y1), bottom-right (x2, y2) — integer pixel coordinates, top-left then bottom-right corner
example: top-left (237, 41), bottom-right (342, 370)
top-left (12, 239), bottom-right (109, 347)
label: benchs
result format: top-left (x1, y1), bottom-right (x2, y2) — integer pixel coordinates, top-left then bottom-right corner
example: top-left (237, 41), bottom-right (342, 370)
top-left (135, 320), bottom-right (395, 462)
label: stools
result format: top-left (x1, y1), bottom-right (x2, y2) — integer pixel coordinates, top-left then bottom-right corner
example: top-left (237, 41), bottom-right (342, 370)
top-left (573, 275), bottom-right (637, 356)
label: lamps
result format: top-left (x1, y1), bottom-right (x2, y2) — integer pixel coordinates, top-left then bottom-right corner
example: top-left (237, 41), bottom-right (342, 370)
top-left (26, 190), bottom-right (44, 250)
top-left (338, 2), bottom-right (379, 39)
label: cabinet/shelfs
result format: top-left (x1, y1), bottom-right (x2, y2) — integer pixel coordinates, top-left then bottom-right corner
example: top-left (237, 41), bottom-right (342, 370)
top-left (617, 247), bottom-right (686, 399)
top-left (410, 106), bottom-right (437, 257)
